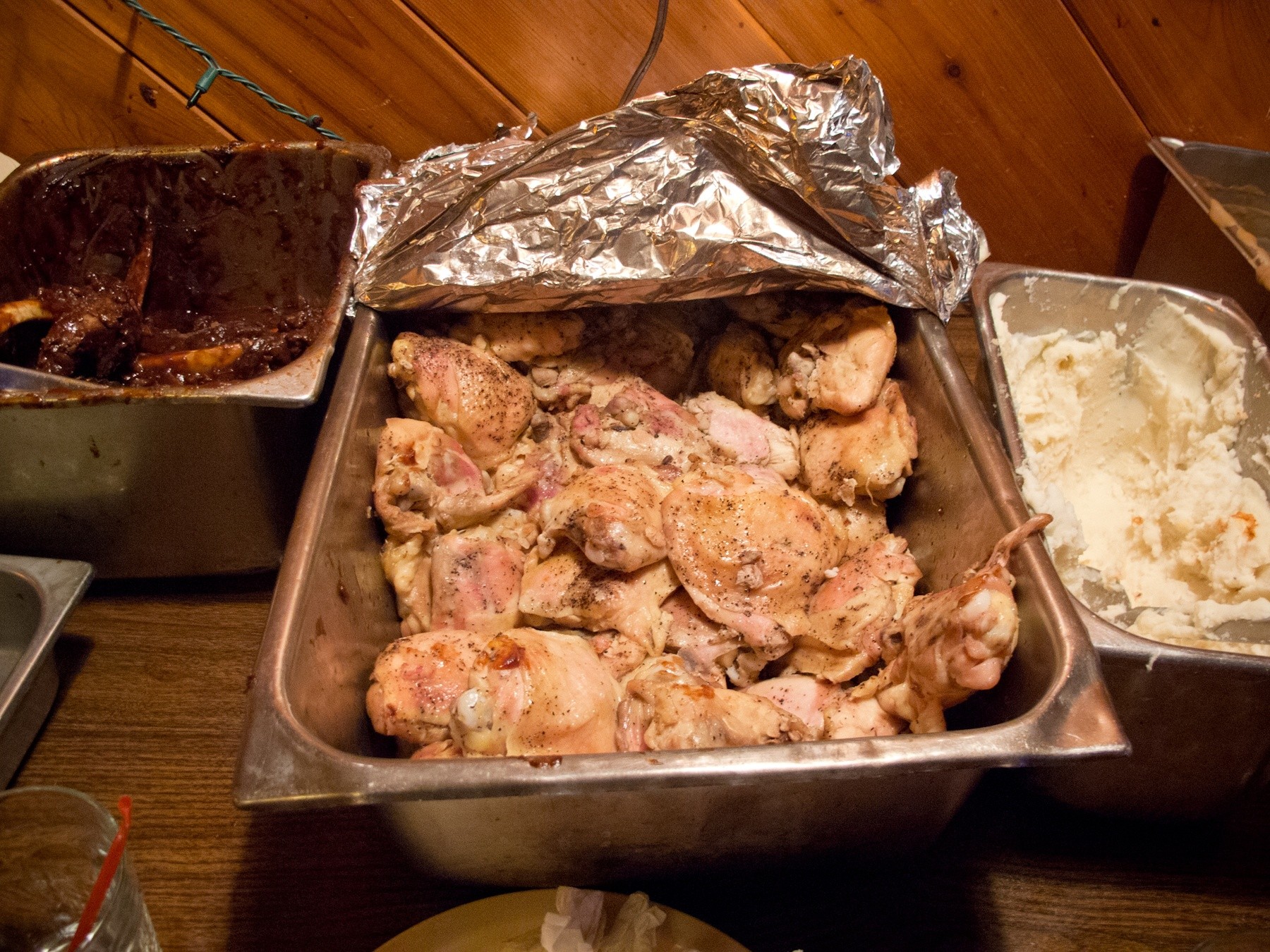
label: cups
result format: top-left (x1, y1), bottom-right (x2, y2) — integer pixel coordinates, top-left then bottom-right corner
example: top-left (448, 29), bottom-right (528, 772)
top-left (0, 786), bottom-right (157, 951)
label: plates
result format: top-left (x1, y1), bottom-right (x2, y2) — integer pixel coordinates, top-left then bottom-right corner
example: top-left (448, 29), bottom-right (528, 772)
top-left (373, 889), bottom-right (749, 952)
